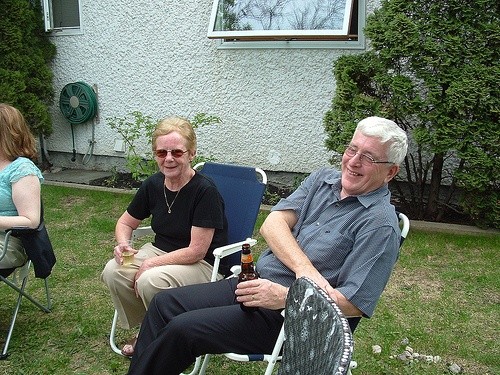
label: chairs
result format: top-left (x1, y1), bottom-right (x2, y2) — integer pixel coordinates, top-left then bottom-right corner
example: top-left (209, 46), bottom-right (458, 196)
top-left (198, 211), bottom-right (410, 375)
top-left (0, 199), bottom-right (55, 361)
top-left (106, 161), bottom-right (268, 375)
top-left (283, 277), bottom-right (354, 375)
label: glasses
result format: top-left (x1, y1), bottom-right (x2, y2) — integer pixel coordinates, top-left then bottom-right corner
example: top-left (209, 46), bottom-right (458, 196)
top-left (343, 144), bottom-right (394, 167)
top-left (154, 148), bottom-right (189, 159)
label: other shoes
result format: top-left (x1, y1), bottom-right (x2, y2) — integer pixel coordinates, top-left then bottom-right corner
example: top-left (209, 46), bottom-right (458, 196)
top-left (120, 336), bottom-right (138, 357)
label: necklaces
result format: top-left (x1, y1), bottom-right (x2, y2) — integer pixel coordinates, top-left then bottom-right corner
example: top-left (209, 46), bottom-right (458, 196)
top-left (164, 180), bottom-right (190, 213)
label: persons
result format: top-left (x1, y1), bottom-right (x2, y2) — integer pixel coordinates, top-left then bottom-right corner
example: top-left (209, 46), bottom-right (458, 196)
top-left (128, 116), bottom-right (408, 375)
top-left (0, 103), bottom-right (44, 270)
top-left (100, 116), bottom-right (233, 357)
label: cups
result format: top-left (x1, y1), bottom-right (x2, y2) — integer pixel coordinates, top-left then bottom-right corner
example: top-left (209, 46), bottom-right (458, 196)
top-left (117, 240), bottom-right (135, 265)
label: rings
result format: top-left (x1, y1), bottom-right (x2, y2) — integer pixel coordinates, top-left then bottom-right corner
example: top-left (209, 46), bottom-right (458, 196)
top-left (252, 295), bottom-right (255, 299)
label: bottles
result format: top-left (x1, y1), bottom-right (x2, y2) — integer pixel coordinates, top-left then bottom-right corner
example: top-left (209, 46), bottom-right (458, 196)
top-left (238, 244), bottom-right (261, 311)
